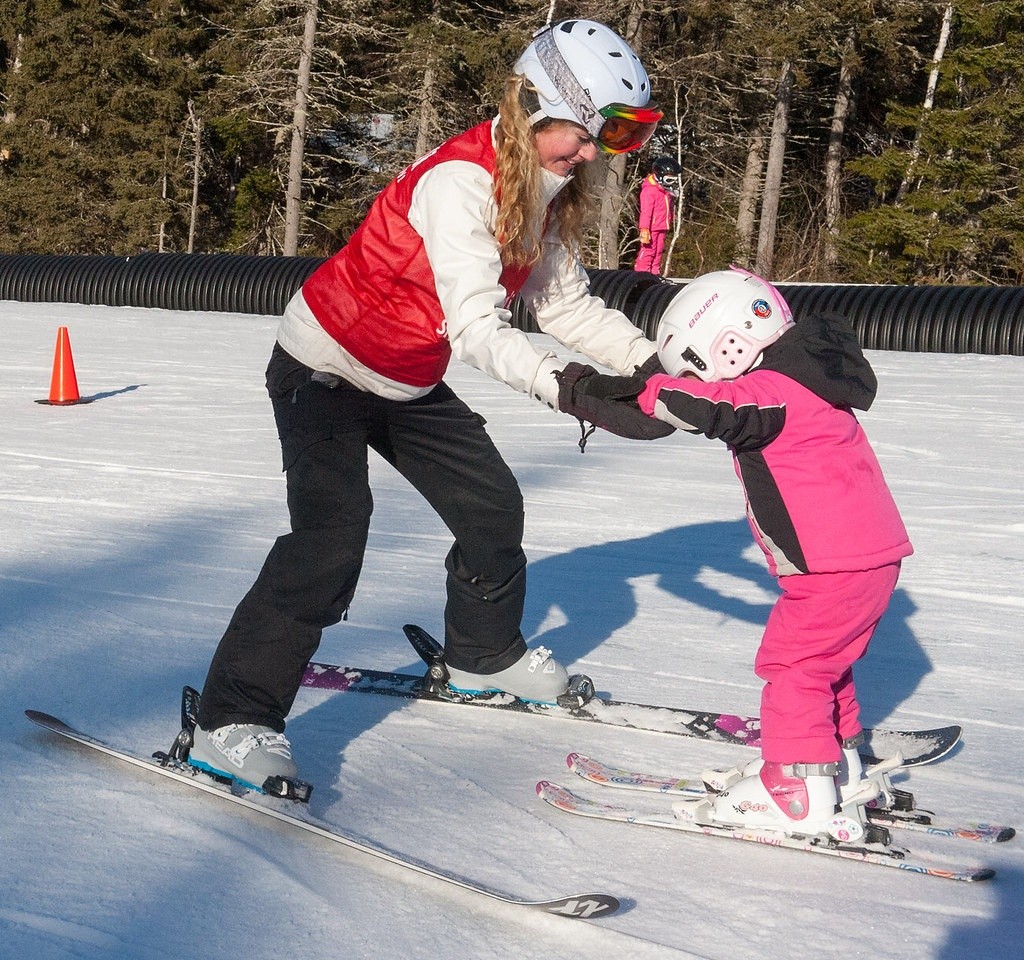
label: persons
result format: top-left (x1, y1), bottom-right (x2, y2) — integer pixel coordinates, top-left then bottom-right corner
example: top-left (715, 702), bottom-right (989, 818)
top-left (184, 21), bottom-right (678, 802)
top-left (634, 157), bottom-right (683, 284)
top-left (636, 265), bottom-right (914, 837)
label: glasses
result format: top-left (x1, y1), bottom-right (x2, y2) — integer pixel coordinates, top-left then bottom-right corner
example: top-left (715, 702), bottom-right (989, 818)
top-left (663, 175), bottom-right (678, 185)
top-left (591, 102), bottom-right (664, 155)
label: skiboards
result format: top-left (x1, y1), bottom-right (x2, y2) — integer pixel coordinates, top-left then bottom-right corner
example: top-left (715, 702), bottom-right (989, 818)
top-left (535, 751), bottom-right (1017, 883)
top-left (21, 658), bottom-right (964, 922)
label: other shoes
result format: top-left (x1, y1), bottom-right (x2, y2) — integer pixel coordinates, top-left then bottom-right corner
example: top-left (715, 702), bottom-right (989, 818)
top-left (746, 747), bottom-right (860, 787)
top-left (712, 774), bottom-right (837, 836)
top-left (193, 723), bottom-right (297, 785)
top-left (445, 649), bottom-right (568, 702)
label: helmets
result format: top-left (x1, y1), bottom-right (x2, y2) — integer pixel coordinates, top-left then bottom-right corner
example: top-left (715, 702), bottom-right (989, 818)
top-left (652, 157), bottom-right (681, 187)
top-left (513, 18), bottom-right (651, 138)
top-left (657, 268), bottom-right (797, 383)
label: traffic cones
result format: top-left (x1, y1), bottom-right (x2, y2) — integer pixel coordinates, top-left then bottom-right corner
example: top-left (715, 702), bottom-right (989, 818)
top-left (32, 325), bottom-right (95, 406)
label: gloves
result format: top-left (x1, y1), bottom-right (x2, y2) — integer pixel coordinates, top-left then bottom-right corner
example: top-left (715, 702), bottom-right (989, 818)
top-left (634, 352), bottom-right (668, 380)
top-left (640, 228), bottom-right (651, 244)
top-left (554, 362), bottom-right (676, 440)
